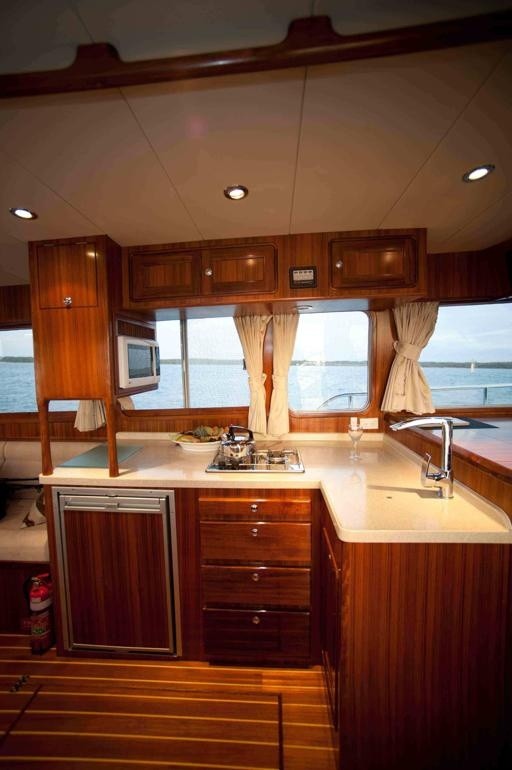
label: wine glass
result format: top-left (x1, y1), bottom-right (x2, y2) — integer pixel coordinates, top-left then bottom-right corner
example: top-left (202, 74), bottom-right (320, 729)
top-left (347, 423), bottom-right (363, 461)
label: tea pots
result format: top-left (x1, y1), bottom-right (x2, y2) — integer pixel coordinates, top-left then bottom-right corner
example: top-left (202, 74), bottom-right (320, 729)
top-left (219, 426), bottom-right (256, 465)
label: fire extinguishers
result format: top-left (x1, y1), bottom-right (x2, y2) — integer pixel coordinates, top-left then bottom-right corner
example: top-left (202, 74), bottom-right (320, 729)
top-left (29, 573), bottom-right (51, 656)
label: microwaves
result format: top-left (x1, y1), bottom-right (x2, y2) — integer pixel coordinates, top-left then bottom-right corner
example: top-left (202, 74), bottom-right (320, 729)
top-left (117, 335), bottom-right (161, 389)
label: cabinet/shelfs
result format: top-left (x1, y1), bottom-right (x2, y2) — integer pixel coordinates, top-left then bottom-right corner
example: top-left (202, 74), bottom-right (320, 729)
top-left (329, 227), bottom-right (427, 299)
top-left (27, 233), bottom-right (158, 478)
top-left (320, 496), bottom-right (512, 770)
top-left (184, 489), bottom-right (322, 666)
top-left (122, 235), bottom-right (277, 310)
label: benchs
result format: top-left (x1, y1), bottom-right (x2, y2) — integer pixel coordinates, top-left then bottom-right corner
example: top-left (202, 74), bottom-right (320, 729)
top-left (0, 441), bottom-right (102, 631)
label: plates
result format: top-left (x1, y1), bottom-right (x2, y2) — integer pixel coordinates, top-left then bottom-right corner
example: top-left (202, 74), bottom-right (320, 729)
top-left (171, 439), bottom-right (223, 451)
top-left (204, 447), bottom-right (305, 474)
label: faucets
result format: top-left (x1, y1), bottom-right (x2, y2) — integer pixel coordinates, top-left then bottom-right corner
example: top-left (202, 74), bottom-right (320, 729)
top-left (390, 415), bottom-right (455, 496)
